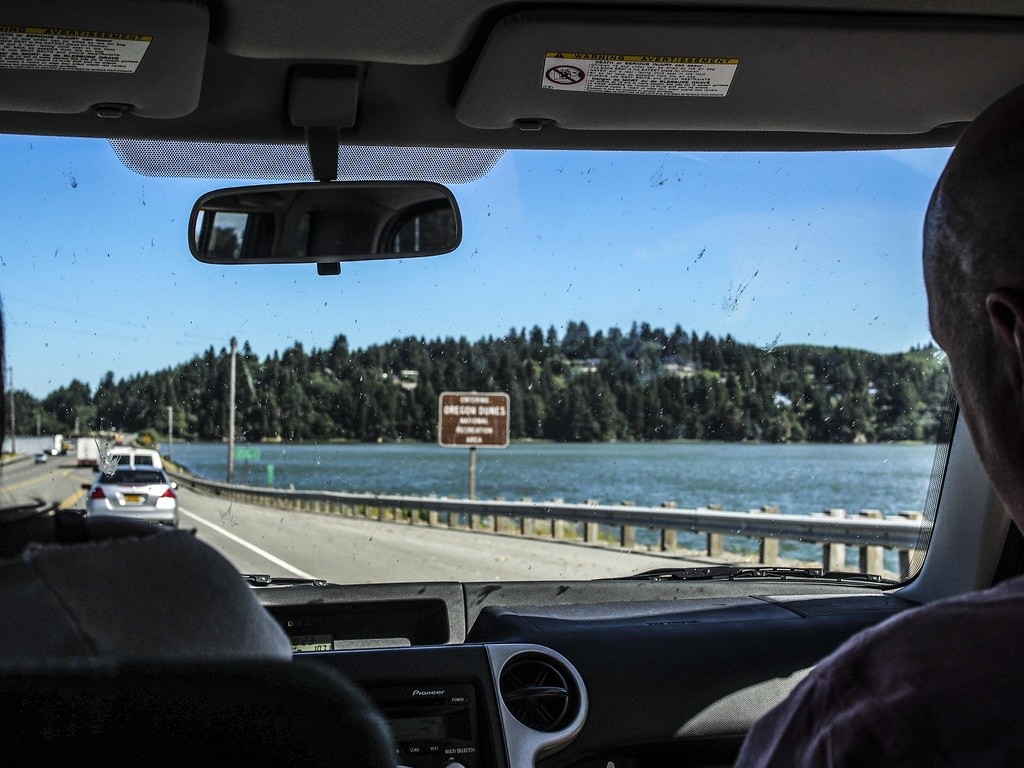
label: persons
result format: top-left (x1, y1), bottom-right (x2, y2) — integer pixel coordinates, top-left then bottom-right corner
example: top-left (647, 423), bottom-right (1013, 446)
top-left (61, 439), bottom-right (72, 455)
top-left (0, 303), bottom-right (294, 677)
top-left (718, 80), bottom-right (1024, 768)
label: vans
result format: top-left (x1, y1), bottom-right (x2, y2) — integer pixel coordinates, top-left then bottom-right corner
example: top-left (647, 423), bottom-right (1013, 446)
top-left (99, 449), bottom-right (164, 472)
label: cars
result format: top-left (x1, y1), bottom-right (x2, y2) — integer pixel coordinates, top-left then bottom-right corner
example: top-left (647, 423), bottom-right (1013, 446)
top-left (33, 454), bottom-right (47, 464)
top-left (83, 464), bottom-right (178, 530)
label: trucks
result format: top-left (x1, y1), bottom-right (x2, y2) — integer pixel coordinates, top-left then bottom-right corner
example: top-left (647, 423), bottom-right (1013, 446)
top-left (75, 435), bottom-right (101, 467)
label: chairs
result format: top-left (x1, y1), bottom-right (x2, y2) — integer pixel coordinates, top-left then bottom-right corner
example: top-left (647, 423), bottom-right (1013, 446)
top-left (3, 650), bottom-right (403, 766)
